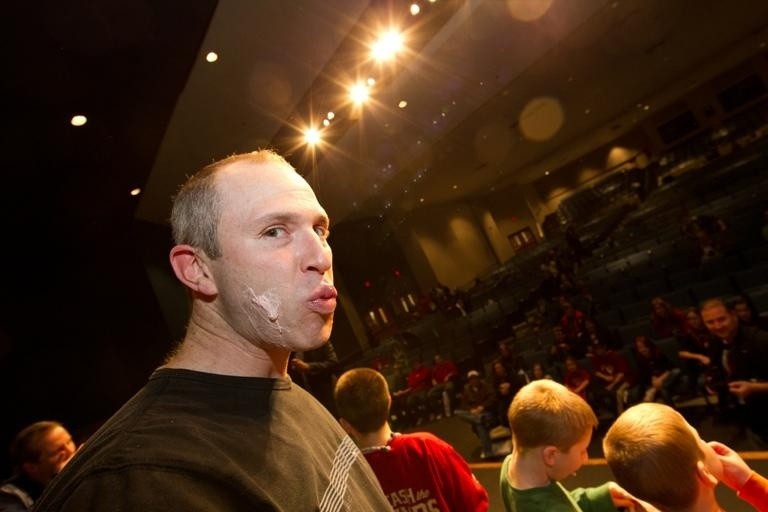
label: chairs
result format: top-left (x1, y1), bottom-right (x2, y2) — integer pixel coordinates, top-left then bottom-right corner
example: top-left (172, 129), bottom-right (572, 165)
top-left (369, 140), bottom-right (767, 404)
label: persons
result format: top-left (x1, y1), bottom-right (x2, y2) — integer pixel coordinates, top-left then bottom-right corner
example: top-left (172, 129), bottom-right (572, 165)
top-left (289, 339), bottom-right (341, 410)
top-left (602, 402), bottom-right (768, 512)
top-left (334, 368), bottom-right (491, 511)
top-left (378, 212), bottom-right (767, 442)
top-left (499, 379), bottom-right (635, 511)
top-left (1, 419), bottom-right (81, 512)
top-left (33, 149), bottom-right (397, 512)
